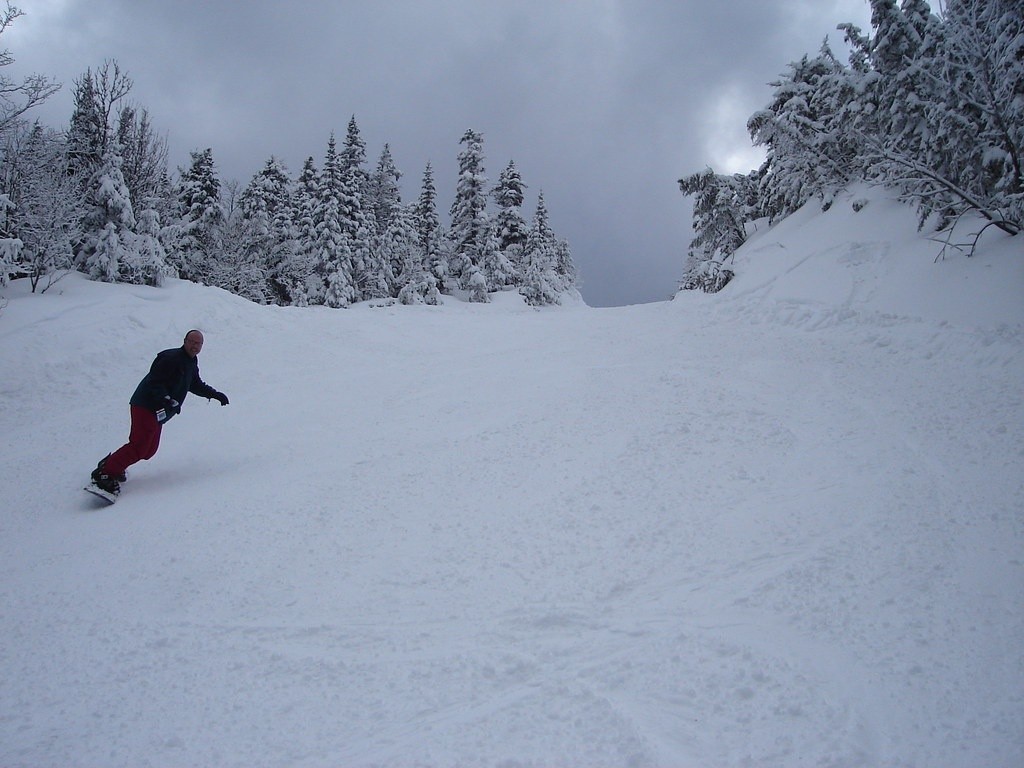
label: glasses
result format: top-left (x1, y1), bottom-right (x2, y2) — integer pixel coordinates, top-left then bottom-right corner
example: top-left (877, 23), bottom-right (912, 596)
top-left (186, 339), bottom-right (203, 345)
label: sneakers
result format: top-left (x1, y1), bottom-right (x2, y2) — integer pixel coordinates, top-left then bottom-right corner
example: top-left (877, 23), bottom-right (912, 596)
top-left (84, 461), bottom-right (121, 504)
top-left (97, 452), bottom-right (128, 483)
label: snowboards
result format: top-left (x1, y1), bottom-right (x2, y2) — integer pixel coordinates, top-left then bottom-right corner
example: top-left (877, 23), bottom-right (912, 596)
top-left (84, 468), bottom-right (128, 503)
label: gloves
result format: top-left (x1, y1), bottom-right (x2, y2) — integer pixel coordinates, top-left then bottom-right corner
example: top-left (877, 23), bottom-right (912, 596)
top-left (214, 392), bottom-right (229, 407)
top-left (170, 399), bottom-right (181, 414)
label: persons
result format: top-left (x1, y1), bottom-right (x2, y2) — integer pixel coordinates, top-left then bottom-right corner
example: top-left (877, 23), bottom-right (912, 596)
top-left (90, 329), bottom-right (230, 495)
